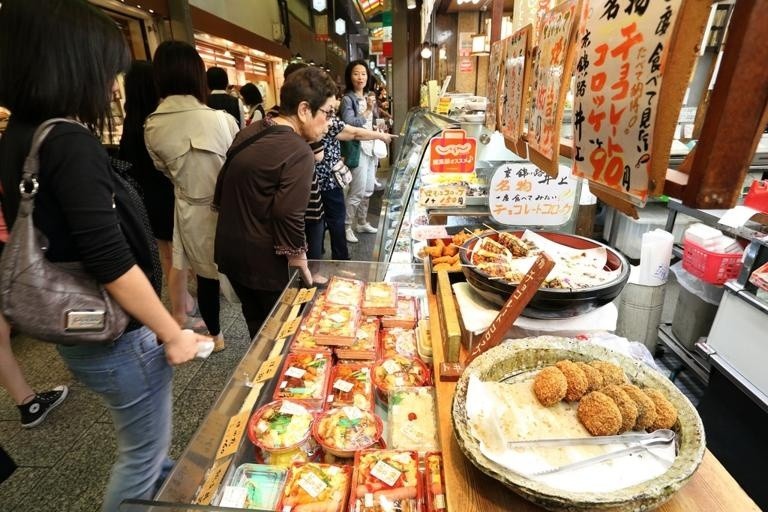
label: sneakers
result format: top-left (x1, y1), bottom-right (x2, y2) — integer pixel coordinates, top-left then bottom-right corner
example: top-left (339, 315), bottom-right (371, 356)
top-left (356, 222), bottom-right (378, 234)
top-left (200, 331), bottom-right (225, 353)
top-left (345, 227), bottom-right (358, 243)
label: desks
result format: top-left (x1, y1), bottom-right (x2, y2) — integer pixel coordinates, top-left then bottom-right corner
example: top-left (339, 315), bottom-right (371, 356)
top-left (425, 281), bottom-right (764, 512)
top-left (651, 320), bottom-right (709, 410)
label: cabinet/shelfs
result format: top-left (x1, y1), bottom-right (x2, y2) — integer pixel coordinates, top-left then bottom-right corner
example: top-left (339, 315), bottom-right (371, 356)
top-left (366, 103), bottom-right (580, 288)
top-left (121, 252), bottom-right (445, 512)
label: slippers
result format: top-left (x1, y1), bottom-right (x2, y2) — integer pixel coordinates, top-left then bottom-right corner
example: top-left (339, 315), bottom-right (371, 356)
top-left (178, 316), bottom-right (208, 331)
top-left (185, 296), bottom-right (199, 317)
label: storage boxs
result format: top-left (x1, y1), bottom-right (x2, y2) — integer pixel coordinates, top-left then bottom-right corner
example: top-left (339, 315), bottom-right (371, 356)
top-left (603, 203), bottom-right (706, 262)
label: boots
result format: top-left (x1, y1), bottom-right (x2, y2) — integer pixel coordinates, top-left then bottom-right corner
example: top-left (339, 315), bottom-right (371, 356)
top-left (16, 383), bottom-right (69, 429)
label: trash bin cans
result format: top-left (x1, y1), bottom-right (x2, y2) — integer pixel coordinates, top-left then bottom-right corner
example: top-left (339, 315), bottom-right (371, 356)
top-left (617, 282), bottom-right (668, 358)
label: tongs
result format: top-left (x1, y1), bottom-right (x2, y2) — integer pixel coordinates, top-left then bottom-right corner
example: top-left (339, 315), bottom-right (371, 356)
top-left (504, 429), bottom-right (677, 481)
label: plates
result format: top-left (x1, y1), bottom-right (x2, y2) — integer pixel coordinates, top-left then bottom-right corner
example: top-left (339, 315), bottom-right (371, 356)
top-left (384, 190), bottom-right (429, 264)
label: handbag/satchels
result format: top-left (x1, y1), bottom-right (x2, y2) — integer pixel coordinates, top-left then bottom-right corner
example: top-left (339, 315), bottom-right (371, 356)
top-left (340, 140), bottom-right (361, 169)
top-left (331, 160), bottom-right (354, 188)
top-left (0, 198), bottom-right (130, 346)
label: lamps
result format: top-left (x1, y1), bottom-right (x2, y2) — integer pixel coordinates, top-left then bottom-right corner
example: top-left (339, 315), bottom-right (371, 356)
top-left (469, 32), bottom-right (492, 59)
top-left (405, 0), bottom-right (417, 9)
top-left (421, 41), bottom-right (438, 59)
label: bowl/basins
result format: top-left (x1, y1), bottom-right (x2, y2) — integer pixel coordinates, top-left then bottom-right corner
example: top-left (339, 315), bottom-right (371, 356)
top-left (451, 332), bottom-right (708, 512)
top-left (457, 226), bottom-right (632, 321)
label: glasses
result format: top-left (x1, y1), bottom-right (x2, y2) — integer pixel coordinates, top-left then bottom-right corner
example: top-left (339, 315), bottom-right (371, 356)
top-left (310, 105), bottom-right (334, 122)
